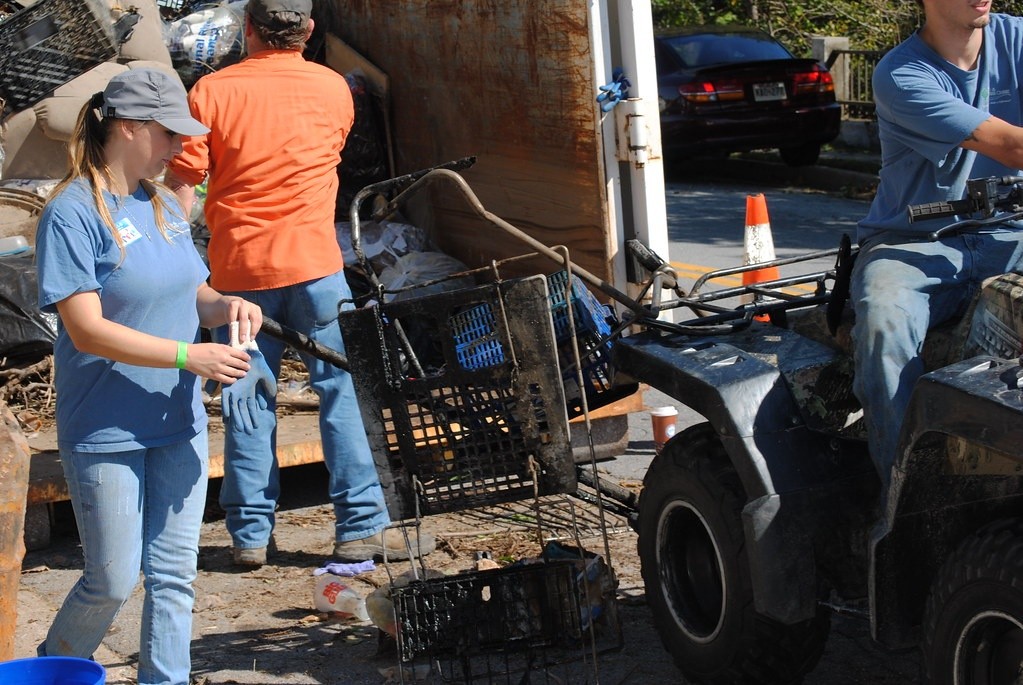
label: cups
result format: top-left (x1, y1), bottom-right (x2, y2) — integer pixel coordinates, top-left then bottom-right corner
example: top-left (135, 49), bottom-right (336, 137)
top-left (650, 406), bottom-right (678, 453)
top-left (314, 576), bottom-right (372, 622)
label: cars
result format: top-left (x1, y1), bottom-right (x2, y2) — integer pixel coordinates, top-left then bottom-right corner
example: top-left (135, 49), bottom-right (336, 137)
top-left (653, 22), bottom-right (842, 169)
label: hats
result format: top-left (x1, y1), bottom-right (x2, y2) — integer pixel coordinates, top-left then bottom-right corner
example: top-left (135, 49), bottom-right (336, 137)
top-left (246, 0), bottom-right (312, 31)
top-left (98, 67), bottom-right (211, 135)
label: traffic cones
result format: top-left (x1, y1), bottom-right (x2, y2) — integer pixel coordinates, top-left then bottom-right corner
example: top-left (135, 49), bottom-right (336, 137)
top-left (741, 193), bottom-right (784, 323)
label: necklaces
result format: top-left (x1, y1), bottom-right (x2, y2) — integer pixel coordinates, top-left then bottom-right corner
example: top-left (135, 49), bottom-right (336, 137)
top-left (100, 176), bottom-right (152, 242)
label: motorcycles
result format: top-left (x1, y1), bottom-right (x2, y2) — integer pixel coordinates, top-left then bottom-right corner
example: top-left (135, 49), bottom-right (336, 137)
top-left (610, 158), bottom-right (1023, 684)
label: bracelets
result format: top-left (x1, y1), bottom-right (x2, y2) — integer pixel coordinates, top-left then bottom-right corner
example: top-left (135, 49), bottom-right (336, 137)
top-left (175, 342), bottom-right (187, 369)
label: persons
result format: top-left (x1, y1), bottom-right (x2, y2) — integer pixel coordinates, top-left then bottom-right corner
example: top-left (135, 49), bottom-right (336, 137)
top-left (35, 70), bottom-right (262, 685)
top-left (163, 0), bottom-right (437, 567)
top-left (832, 0), bottom-right (1023, 605)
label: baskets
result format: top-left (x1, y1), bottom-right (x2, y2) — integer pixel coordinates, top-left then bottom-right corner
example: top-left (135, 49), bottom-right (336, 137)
top-left (448, 268), bottom-right (615, 377)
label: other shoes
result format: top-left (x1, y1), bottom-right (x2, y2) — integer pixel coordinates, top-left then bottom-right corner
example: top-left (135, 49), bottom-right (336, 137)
top-left (839, 570), bottom-right (869, 606)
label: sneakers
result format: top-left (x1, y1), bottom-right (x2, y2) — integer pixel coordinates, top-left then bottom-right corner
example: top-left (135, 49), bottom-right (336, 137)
top-left (232, 537), bottom-right (281, 568)
top-left (332, 528), bottom-right (435, 561)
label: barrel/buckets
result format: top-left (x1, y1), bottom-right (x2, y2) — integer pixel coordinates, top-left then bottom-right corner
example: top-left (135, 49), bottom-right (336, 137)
top-left (1, 656), bottom-right (106, 685)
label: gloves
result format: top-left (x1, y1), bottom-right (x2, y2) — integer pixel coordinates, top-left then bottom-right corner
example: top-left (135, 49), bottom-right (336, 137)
top-left (596, 68), bottom-right (632, 112)
top-left (205, 320), bottom-right (277, 435)
